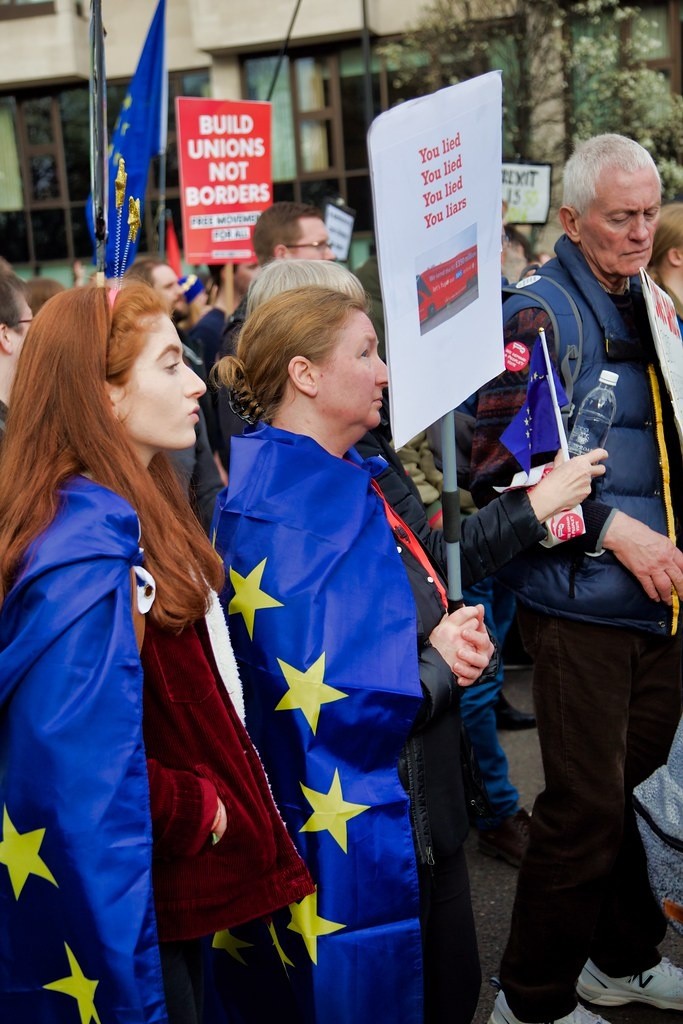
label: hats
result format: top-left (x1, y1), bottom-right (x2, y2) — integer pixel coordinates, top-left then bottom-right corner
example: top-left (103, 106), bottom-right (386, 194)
top-left (178, 274), bottom-right (205, 304)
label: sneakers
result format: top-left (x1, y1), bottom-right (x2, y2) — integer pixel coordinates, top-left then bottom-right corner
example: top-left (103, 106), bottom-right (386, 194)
top-left (478, 808), bottom-right (532, 867)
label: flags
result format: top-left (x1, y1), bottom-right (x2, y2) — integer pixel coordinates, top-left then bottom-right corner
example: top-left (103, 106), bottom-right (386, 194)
top-left (84, 1), bottom-right (165, 277)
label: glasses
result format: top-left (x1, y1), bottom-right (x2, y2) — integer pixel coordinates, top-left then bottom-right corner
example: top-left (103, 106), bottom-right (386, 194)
top-left (286, 240), bottom-right (332, 251)
top-left (502, 235), bottom-right (510, 243)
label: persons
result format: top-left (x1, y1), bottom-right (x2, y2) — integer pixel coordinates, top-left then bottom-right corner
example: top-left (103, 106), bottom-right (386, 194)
top-left (0, 134), bottom-right (683, 1024)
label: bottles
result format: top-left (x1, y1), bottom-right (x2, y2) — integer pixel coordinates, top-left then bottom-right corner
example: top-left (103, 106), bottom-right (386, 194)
top-left (568, 370), bottom-right (620, 479)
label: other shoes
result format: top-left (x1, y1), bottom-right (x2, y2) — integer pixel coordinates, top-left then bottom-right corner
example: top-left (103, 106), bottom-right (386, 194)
top-left (485, 976), bottom-right (612, 1024)
top-left (491, 692), bottom-right (536, 730)
top-left (575, 956), bottom-right (683, 1010)
